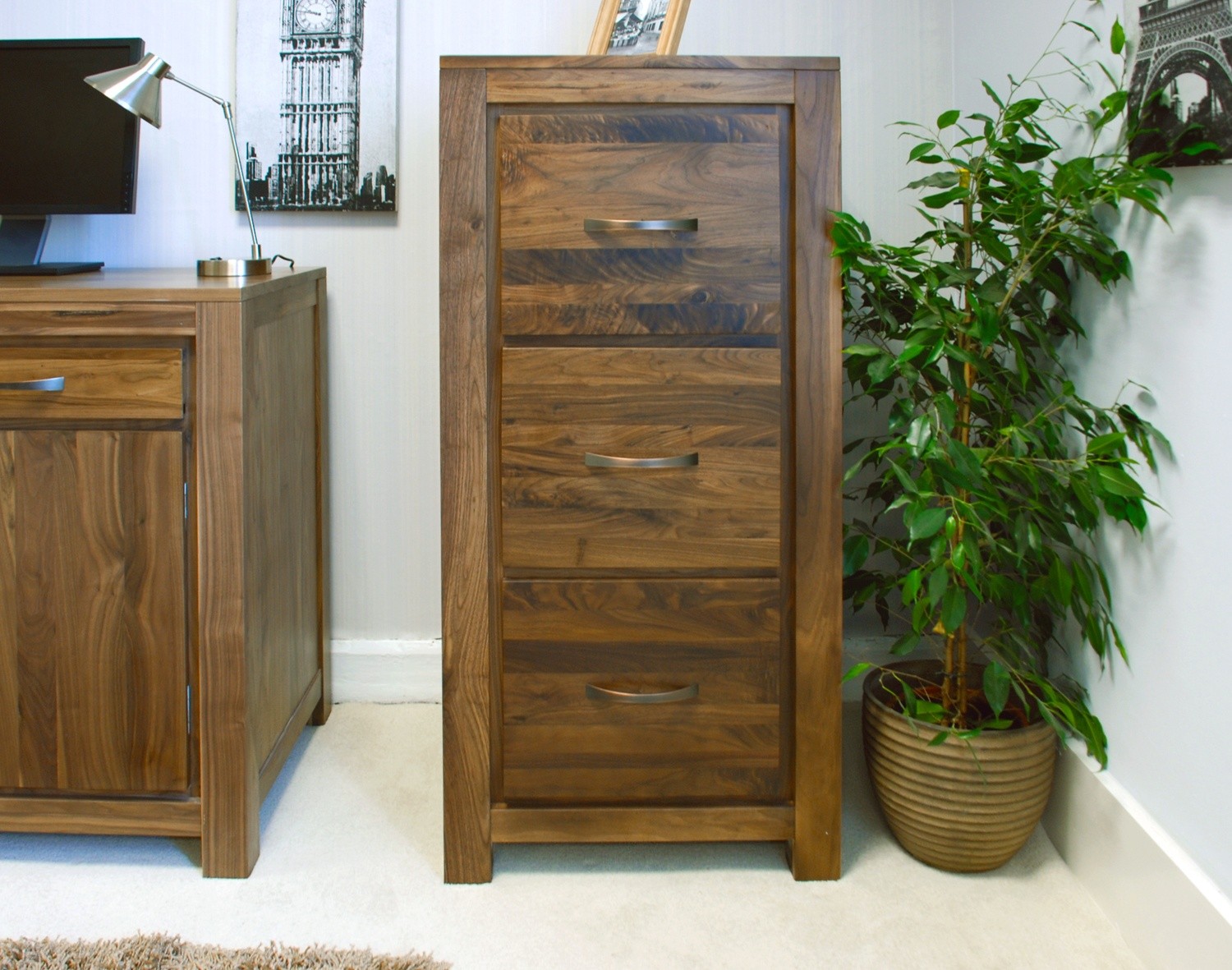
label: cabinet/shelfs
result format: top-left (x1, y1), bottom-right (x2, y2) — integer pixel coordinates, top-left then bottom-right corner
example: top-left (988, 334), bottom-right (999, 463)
top-left (0, 265), bottom-right (337, 879)
top-left (437, 52), bottom-right (845, 886)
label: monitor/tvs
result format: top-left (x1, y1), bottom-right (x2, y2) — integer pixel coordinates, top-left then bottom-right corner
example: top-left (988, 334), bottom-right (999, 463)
top-left (0, 37), bottom-right (145, 278)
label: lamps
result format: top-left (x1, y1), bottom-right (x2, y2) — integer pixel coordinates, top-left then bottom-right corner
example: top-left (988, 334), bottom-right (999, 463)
top-left (84, 52), bottom-right (276, 278)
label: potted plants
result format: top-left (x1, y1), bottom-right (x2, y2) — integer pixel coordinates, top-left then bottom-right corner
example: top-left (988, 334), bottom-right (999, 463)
top-left (827, 0), bottom-right (1226, 878)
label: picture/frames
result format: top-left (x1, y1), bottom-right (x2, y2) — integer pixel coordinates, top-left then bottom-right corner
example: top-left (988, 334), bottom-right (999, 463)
top-left (586, 0), bottom-right (689, 54)
top-left (229, 0), bottom-right (400, 216)
